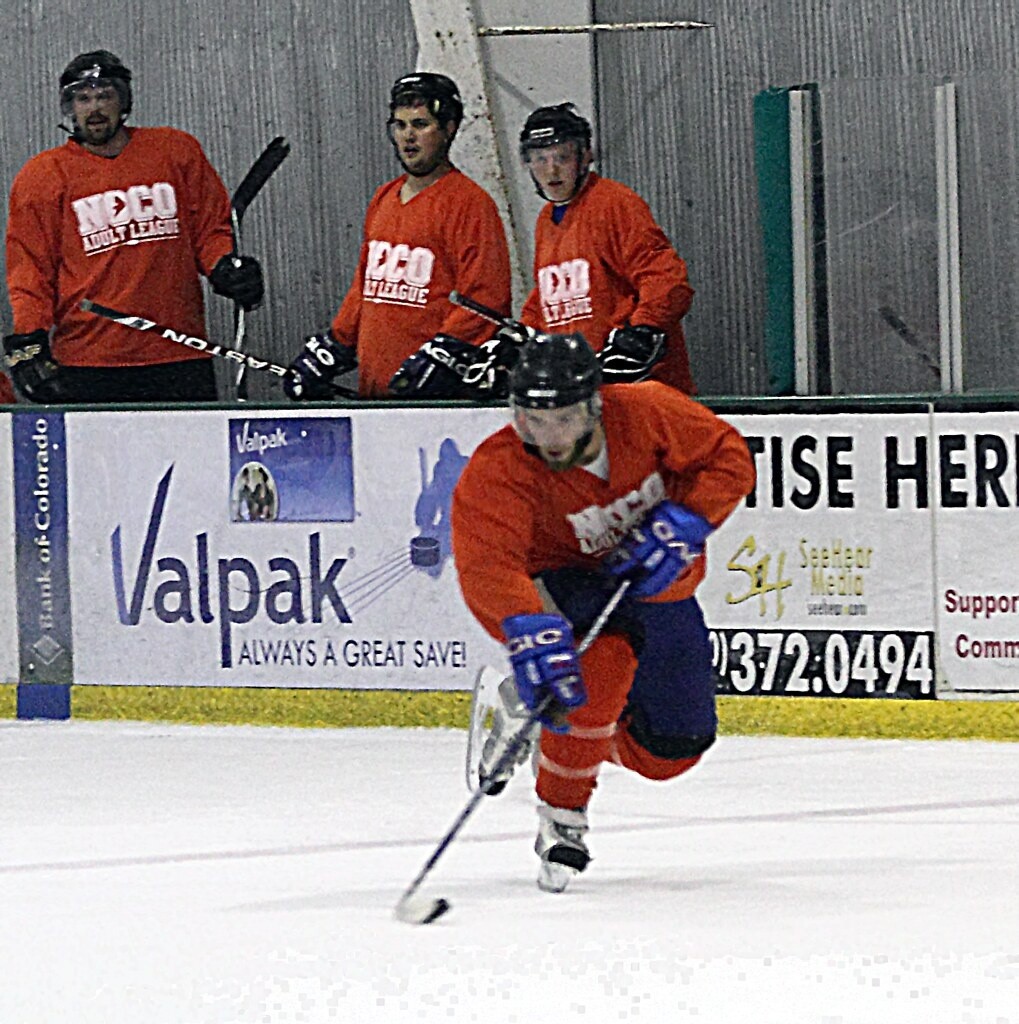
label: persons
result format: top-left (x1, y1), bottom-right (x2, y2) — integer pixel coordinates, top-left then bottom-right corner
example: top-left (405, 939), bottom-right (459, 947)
top-left (514, 102), bottom-right (695, 400)
top-left (448, 332), bottom-right (757, 875)
top-left (285, 73), bottom-right (510, 412)
top-left (7, 47), bottom-right (270, 406)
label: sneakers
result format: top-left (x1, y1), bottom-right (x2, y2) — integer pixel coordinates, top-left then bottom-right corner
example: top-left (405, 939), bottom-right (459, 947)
top-left (464, 662), bottom-right (541, 798)
top-left (533, 802), bottom-right (590, 894)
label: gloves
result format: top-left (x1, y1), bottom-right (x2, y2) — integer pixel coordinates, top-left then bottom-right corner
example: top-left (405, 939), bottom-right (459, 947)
top-left (505, 617), bottom-right (586, 736)
top-left (212, 254), bottom-right (264, 312)
top-left (601, 501), bottom-right (713, 602)
top-left (3, 329), bottom-right (80, 405)
top-left (387, 334), bottom-right (482, 400)
top-left (593, 326), bottom-right (668, 389)
top-left (281, 330), bottom-right (358, 400)
top-left (459, 320), bottom-right (529, 402)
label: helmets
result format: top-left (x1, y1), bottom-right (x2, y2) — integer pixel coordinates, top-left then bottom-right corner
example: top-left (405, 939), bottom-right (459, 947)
top-left (389, 72), bottom-right (464, 123)
top-left (520, 102), bottom-right (591, 153)
top-left (58, 49), bottom-right (132, 116)
top-left (510, 330), bottom-right (601, 408)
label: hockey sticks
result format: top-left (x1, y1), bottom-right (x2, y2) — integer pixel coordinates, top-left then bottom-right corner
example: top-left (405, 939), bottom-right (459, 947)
top-left (391, 576), bottom-right (634, 928)
top-left (226, 134), bottom-right (293, 405)
top-left (78, 297), bottom-right (361, 402)
top-left (448, 290), bottom-right (547, 340)
